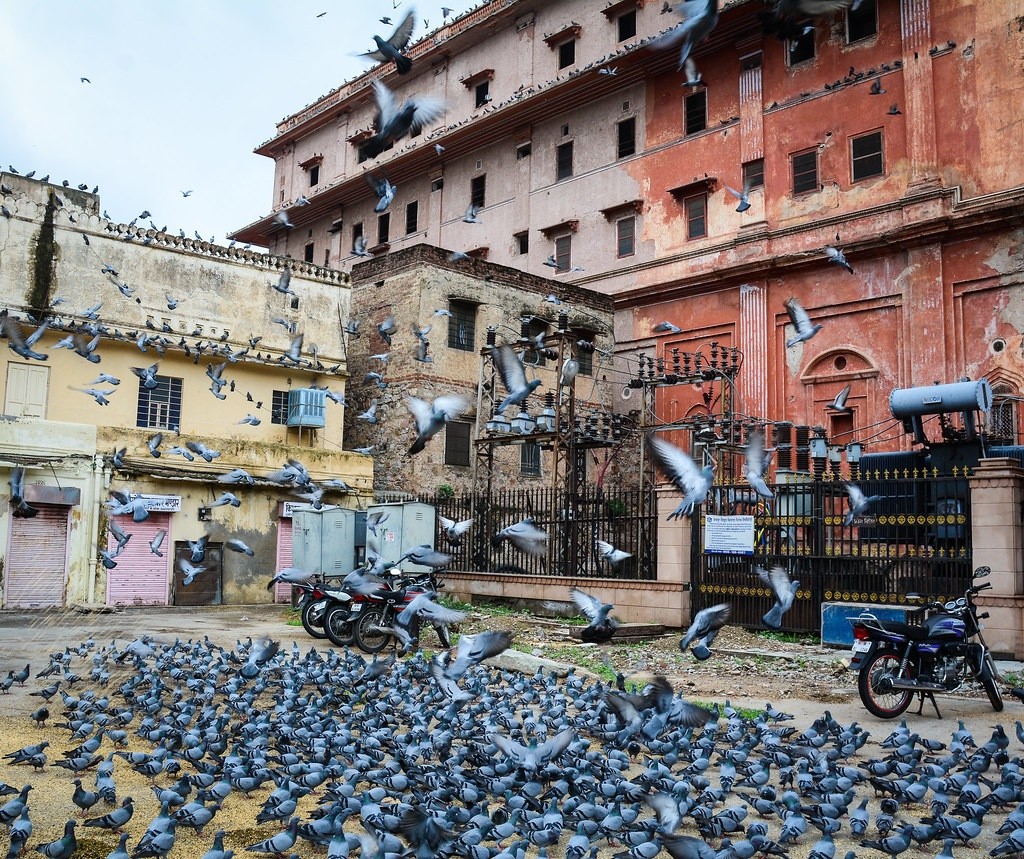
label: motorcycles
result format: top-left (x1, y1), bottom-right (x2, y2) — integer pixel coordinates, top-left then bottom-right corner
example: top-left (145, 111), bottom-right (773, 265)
top-left (285, 544), bottom-right (453, 656)
top-left (844, 565), bottom-right (1006, 722)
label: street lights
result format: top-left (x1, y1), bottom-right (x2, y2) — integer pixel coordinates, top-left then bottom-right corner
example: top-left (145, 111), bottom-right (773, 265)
top-left (538, 356), bottom-right (580, 575)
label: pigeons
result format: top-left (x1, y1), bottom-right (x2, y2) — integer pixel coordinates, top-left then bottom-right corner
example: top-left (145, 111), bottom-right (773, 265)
top-left (0, 2), bottom-right (1023, 858)
